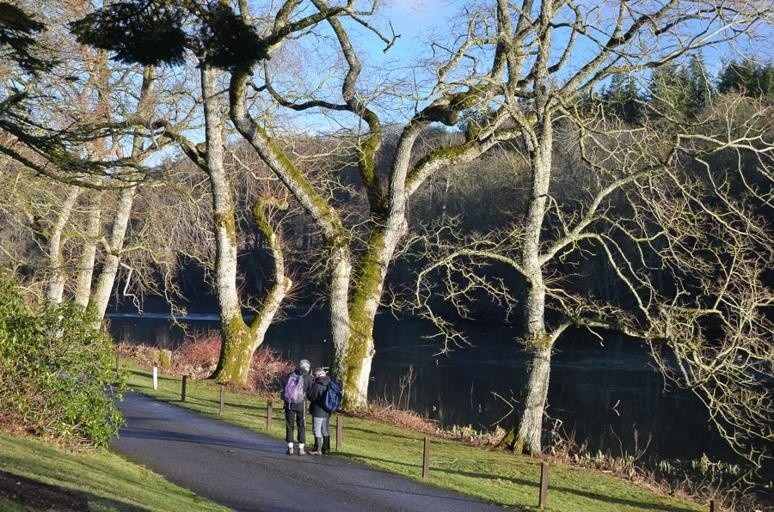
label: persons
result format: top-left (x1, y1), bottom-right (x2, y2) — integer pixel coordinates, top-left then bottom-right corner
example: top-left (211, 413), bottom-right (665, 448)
top-left (280, 359), bottom-right (312, 456)
top-left (306, 367), bottom-right (332, 456)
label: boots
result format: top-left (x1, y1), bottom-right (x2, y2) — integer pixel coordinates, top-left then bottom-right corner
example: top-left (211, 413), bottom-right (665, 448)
top-left (309, 436), bottom-right (330, 455)
top-left (298, 444), bottom-right (306, 455)
top-left (286, 443), bottom-right (293, 455)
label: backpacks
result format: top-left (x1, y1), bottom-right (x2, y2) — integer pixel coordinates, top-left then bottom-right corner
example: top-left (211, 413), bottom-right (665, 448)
top-left (283, 373), bottom-right (307, 404)
top-left (317, 382), bottom-right (341, 413)
